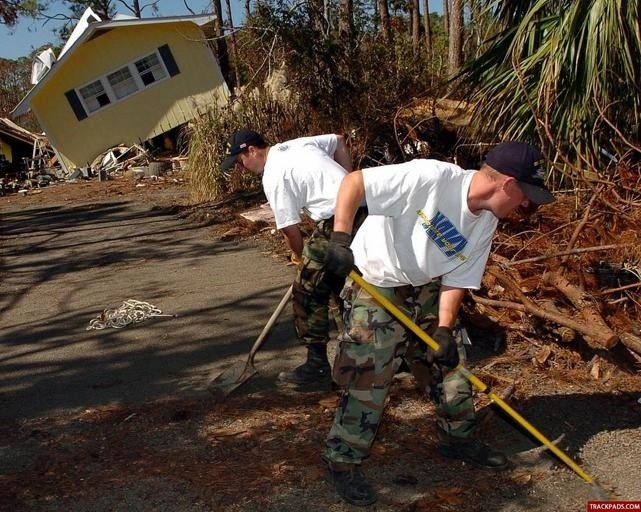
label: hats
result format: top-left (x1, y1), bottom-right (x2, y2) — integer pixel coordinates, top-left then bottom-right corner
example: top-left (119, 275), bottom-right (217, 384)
top-left (485, 142), bottom-right (557, 205)
top-left (221, 128), bottom-right (260, 169)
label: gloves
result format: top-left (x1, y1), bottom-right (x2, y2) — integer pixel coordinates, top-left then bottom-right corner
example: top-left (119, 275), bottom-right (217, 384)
top-left (321, 231), bottom-right (354, 283)
top-left (425, 327), bottom-right (459, 369)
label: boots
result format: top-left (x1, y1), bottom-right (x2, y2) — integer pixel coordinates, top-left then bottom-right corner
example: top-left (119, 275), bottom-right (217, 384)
top-left (277, 342), bottom-right (330, 384)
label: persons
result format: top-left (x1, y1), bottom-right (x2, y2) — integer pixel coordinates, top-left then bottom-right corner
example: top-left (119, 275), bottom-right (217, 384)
top-left (316, 137), bottom-right (556, 507)
top-left (216, 128), bottom-right (440, 401)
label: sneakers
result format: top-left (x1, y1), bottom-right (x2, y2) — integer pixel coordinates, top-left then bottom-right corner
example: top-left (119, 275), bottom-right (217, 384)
top-left (321, 456), bottom-right (377, 506)
top-left (439, 436), bottom-right (509, 472)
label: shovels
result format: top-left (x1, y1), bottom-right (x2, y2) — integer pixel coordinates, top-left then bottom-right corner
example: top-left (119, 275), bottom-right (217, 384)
top-left (207, 239), bottom-right (313, 401)
